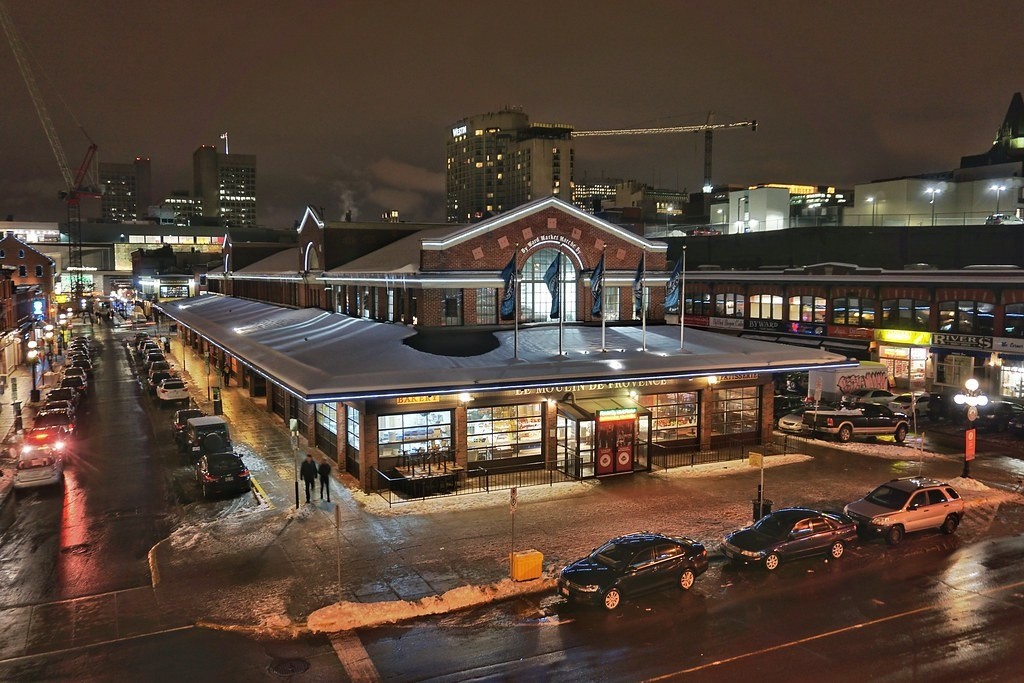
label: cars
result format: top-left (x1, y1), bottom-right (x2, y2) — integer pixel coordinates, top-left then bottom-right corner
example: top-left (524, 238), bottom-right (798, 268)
top-left (777, 386), bottom-right (1024, 443)
top-left (557, 531), bottom-right (709, 612)
top-left (133, 331), bottom-right (190, 405)
top-left (173, 409), bottom-right (208, 437)
top-left (13, 336), bottom-right (92, 490)
top-left (720, 506), bottom-right (858, 574)
top-left (195, 451), bottom-right (252, 499)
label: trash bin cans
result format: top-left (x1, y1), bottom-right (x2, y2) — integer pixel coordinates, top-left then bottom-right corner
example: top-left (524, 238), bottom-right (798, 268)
top-left (751, 499), bottom-right (774, 524)
top-left (509, 549), bottom-right (543, 582)
top-left (158, 338), bottom-right (165, 349)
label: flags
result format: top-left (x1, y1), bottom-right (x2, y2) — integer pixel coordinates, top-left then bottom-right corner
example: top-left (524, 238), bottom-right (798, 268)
top-left (543, 253), bottom-right (560, 318)
top-left (588, 255), bottom-right (603, 318)
top-left (663, 255), bottom-right (683, 309)
top-left (632, 255), bottom-right (643, 317)
top-left (500, 253), bottom-right (515, 320)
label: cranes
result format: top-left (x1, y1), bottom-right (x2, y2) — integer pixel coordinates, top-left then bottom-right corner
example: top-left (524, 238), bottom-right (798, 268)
top-left (570, 111), bottom-right (758, 194)
top-left (1, 6), bottom-right (103, 206)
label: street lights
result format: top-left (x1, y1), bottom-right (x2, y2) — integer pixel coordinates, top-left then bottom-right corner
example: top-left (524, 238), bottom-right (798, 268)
top-left (27, 307), bottom-right (75, 402)
top-left (953, 379), bottom-right (988, 477)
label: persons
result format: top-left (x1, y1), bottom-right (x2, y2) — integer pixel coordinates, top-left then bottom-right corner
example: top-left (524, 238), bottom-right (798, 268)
top-left (221, 365), bottom-right (231, 386)
top-left (300, 453), bottom-right (317, 503)
top-left (107, 312), bottom-right (110, 318)
top-left (110, 310), bottom-right (114, 320)
top-left (317, 457), bottom-right (331, 502)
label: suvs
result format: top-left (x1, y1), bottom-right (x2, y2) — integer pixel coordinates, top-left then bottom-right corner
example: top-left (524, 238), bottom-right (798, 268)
top-left (184, 415), bottom-right (234, 459)
top-left (847, 474), bottom-right (965, 545)
